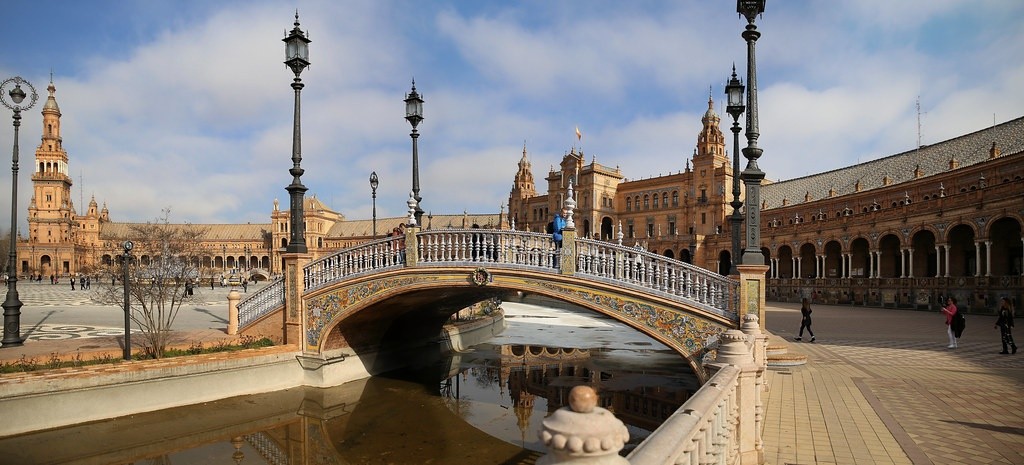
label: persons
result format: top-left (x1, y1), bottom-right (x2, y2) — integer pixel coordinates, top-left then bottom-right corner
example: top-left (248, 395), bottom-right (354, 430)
top-left (186, 279), bottom-right (193, 296)
top-left (940, 297), bottom-right (965, 349)
top-left (151, 274), bottom-right (179, 288)
top-left (552, 208), bottom-right (567, 267)
top-left (594, 233), bottom-right (600, 241)
top-left (30, 274), bottom-right (42, 283)
top-left (995, 297), bottom-right (1018, 354)
top-left (220, 275), bottom-right (228, 288)
top-left (80, 275), bottom-right (90, 290)
top-left (70, 275), bottom-right (76, 290)
top-left (391, 222), bottom-right (421, 262)
top-left (50, 274), bottom-right (60, 285)
top-left (211, 276), bottom-right (214, 290)
top-left (471, 223), bottom-right (484, 261)
top-left (794, 298), bottom-right (815, 342)
top-left (243, 279), bottom-right (248, 292)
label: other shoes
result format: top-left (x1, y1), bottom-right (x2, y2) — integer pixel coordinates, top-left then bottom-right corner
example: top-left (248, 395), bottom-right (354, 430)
top-left (794, 337), bottom-right (802, 342)
top-left (999, 350), bottom-right (1010, 354)
top-left (1011, 346), bottom-right (1017, 354)
top-left (810, 337), bottom-right (815, 342)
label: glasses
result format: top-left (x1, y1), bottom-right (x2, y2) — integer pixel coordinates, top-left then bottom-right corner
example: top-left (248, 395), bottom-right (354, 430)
top-left (947, 300), bottom-right (953, 301)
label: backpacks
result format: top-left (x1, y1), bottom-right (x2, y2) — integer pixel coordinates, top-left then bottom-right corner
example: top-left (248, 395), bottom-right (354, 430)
top-left (546, 222), bottom-right (554, 234)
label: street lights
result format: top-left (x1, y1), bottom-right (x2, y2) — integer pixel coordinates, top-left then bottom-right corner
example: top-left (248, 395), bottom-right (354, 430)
top-left (402, 78), bottom-right (427, 258)
top-left (282, 9), bottom-right (312, 254)
top-left (369, 173), bottom-right (379, 239)
top-left (122, 240), bottom-right (134, 359)
top-left (723, 62), bottom-right (747, 276)
top-left (0, 76), bottom-right (38, 348)
top-left (734, 0), bottom-right (764, 265)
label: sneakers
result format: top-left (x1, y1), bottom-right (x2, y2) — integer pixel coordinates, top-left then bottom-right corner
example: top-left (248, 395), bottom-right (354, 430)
top-left (948, 344), bottom-right (958, 349)
